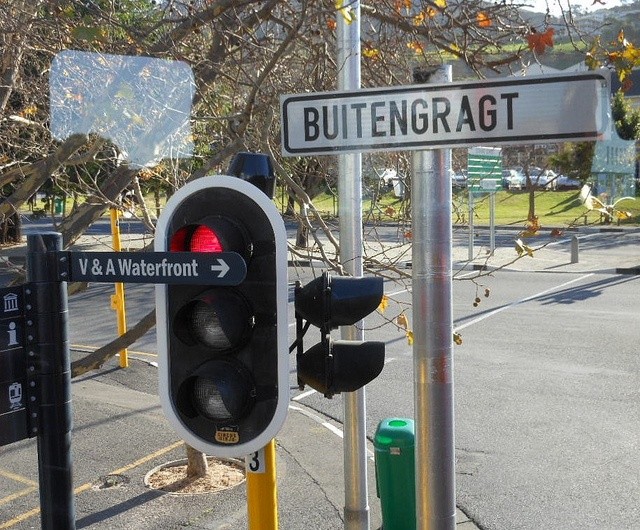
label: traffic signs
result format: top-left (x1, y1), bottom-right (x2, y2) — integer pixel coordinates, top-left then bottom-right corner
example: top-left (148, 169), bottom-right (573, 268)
top-left (67, 249), bottom-right (248, 285)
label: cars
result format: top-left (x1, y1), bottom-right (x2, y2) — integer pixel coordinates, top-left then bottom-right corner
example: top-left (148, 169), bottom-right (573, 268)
top-left (555, 169), bottom-right (585, 190)
top-left (452, 170), bottom-right (468, 188)
top-left (521, 169), bottom-right (557, 189)
top-left (501, 169), bottom-right (523, 188)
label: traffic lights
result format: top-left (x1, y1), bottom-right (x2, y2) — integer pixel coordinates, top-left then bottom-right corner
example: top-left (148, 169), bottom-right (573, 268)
top-left (156, 151), bottom-right (289, 460)
top-left (294, 272), bottom-right (386, 400)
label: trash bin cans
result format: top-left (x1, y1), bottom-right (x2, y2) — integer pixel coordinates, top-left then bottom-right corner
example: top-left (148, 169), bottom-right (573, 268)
top-left (375, 418), bottom-right (416, 530)
